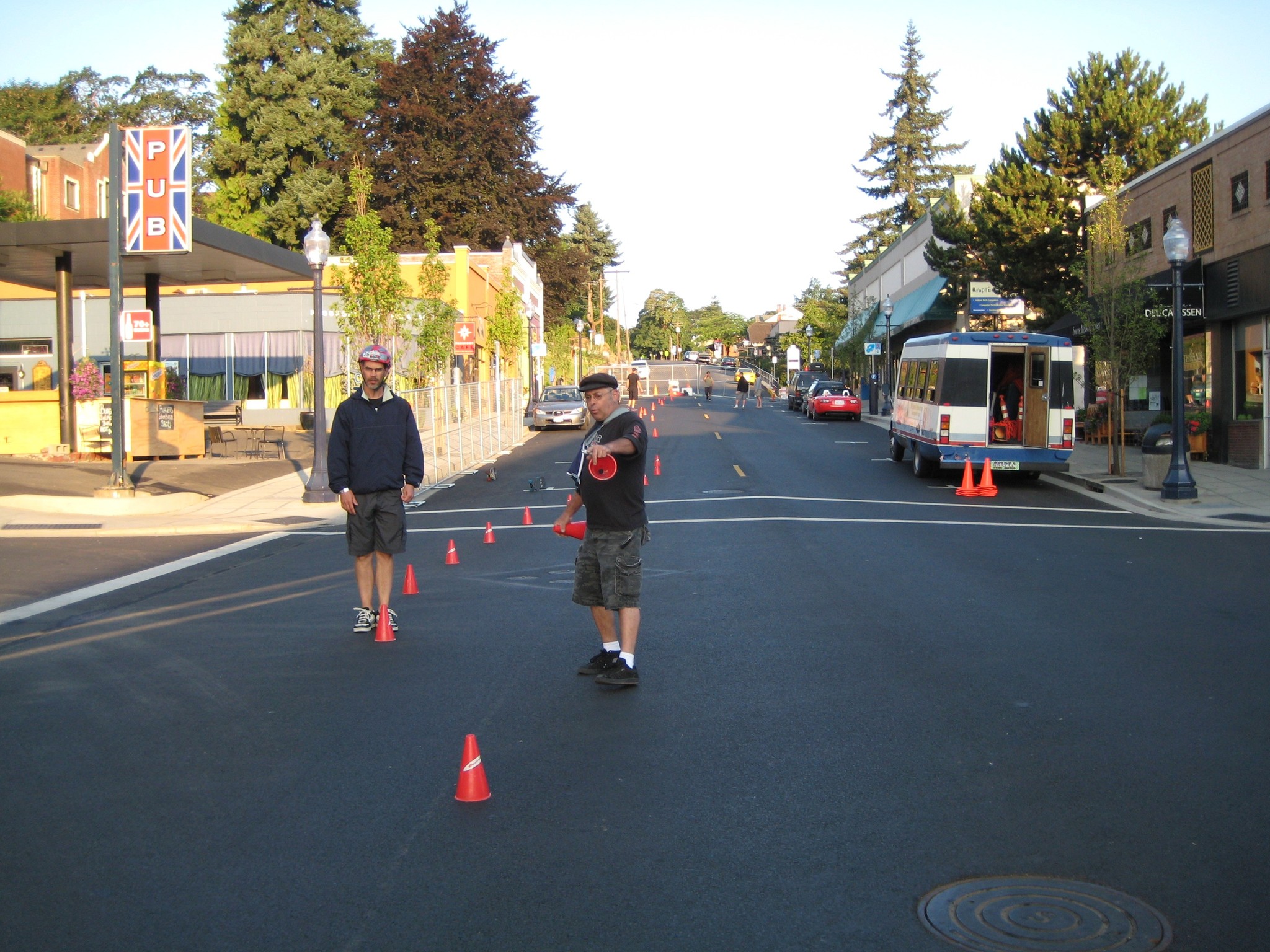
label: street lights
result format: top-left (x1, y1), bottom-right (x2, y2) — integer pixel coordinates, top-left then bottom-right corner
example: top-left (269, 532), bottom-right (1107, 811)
top-left (303, 213), bottom-right (339, 504)
top-left (524, 299), bottom-right (536, 417)
top-left (576, 317), bottom-right (584, 382)
top-left (882, 295), bottom-right (894, 415)
top-left (805, 324), bottom-right (813, 365)
top-left (1163, 218), bottom-right (1200, 499)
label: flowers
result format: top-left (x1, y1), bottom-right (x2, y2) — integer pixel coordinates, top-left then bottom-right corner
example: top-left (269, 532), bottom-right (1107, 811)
top-left (164, 365), bottom-right (185, 400)
top-left (70, 357), bottom-right (104, 400)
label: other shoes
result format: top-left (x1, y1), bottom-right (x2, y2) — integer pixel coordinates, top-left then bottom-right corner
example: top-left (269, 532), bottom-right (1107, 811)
top-left (741, 407), bottom-right (744, 409)
top-left (758, 406), bottom-right (762, 408)
top-left (709, 398), bottom-right (711, 401)
top-left (706, 396), bottom-right (708, 399)
top-left (755, 405), bottom-right (759, 408)
top-left (734, 406), bottom-right (738, 409)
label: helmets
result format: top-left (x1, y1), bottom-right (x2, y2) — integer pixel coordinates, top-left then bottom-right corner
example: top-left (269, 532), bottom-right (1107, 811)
top-left (358, 344), bottom-right (391, 368)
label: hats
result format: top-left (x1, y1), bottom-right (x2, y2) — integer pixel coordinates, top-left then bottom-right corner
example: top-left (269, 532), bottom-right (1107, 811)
top-left (632, 368), bottom-right (637, 371)
top-left (578, 373), bottom-right (618, 392)
top-left (756, 373), bottom-right (759, 377)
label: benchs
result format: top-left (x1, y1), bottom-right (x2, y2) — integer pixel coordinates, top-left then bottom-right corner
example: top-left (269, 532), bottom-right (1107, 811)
top-left (204, 400), bottom-right (243, 425)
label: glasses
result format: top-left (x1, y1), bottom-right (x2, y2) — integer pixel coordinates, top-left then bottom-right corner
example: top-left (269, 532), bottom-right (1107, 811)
top-left (582, 389), bottom-right (614, 403)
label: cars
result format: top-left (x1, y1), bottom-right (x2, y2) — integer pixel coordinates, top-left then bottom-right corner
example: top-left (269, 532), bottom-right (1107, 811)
top-left (720, 357), bottom-right (736, 371)
top-left (531, 385), bottom-right (590, 431)
top-left (801, 379), bottom-right (852, 418)
top-left (629, 360), bottom-right (650, 378)
top-left (734, 367), bottom-right (757, 385)
top-left (684, 351), bottom-right (711, 365)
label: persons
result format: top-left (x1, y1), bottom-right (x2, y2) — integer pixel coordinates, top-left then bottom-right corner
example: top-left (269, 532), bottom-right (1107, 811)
top-left (626, 368), bottom-right (644, 411)
top-left (327, 345), bottom-right (424, 632)
top-left (553, 373), bottom-right (651, 685)
top-left (703, 371), bottom-right (714, 401)
top-left (754, 373), bottom-right (763, 408)
top-left (732, 372), bottom-right (749, 409)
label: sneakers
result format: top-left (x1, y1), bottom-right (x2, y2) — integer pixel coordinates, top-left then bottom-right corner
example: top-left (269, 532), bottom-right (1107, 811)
top-left (627, 406), bottom-right (631, 410)
top-left (631, 407), bottom-right (638, 411)
top-left (375, 608), bottom-right (398, 631)
top-left (578, 649), bottom-right (619, 674)
top-left (352, 607), bottom-right (376, 632)
top-left (595, 657), bottom-right (639, 684)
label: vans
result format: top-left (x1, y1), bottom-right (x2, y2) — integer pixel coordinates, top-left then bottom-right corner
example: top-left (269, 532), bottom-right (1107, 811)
top-left (889, 332), bottom-right (1076, 479)
top-left (785, 362), bottom-right (830, 411)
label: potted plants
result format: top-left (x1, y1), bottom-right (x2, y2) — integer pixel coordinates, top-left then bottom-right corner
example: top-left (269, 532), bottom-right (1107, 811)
top-left (300, 356), bottom-right (317, 429)
top-left (1145, 411), bottom-right (1212, 462)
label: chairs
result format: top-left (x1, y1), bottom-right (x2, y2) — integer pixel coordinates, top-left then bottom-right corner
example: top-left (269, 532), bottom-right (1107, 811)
top-left (257, 425), bottom-right (285, 459)
top-left (548, 393), bottom-right (557, 400)
top-left (822, 389), bottom-right (832, 396)
top-left (207, 426), bottom-right (237, 459)
top-left (842, 390), bottom-right (850, 396)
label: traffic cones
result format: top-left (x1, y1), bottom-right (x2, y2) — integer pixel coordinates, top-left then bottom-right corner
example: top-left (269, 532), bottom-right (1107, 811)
top-left (650, 414), bottom-right (655, 422)
top-left (660, 400), bottom-right (664, 406)
top-left (483, 522), bottom-right (496, 543)
top-left (651, 402), bottom-right (655, 411)
top-left (654, 455), bottom-right (661, 476)
top-left (375, 604), bottom-right (396, 641)
top-left (523, 506), bottom-right (533, 525)
top-left (402, 564), bottom-right (419, 594)
top-left (445, 539), bottom-right (460, 564)
top-left (653, 428), bottom-right (658, 437)
top-left (553, 521), bottom-right (587, 540)
top-left (639, 406), bottom-right (647, 419)
top-left (454, 734), bottom-right (492, 803)
top-left (670, 397), bottom-right (673, 401)
top-left (955, 458), bottom-right (997, 497)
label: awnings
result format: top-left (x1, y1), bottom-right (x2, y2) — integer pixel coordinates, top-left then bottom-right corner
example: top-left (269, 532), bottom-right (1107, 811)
top-left (832, 301), bottom-right (880, 357)
top-left (869, 275), bottom-right (959, 346)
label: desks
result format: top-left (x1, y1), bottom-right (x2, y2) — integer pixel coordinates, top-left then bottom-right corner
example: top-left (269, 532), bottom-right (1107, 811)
top-left (234, 427), bottom-right (275, 459)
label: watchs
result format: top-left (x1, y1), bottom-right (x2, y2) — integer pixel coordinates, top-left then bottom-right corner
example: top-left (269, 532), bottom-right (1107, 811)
top-left (338, 487), bottom-right (349, 495)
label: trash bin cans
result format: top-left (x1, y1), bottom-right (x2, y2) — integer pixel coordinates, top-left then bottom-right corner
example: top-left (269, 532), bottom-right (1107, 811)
top-left (1141, 425), bottom-right (1191, 491)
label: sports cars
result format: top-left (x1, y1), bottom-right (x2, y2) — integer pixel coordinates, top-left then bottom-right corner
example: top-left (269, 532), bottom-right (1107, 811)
top-left (809, 387), bottom-right (861, 421)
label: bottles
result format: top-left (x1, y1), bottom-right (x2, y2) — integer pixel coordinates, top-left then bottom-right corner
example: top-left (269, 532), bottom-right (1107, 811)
top-left (124, 385), bottom-right (133, 394)
